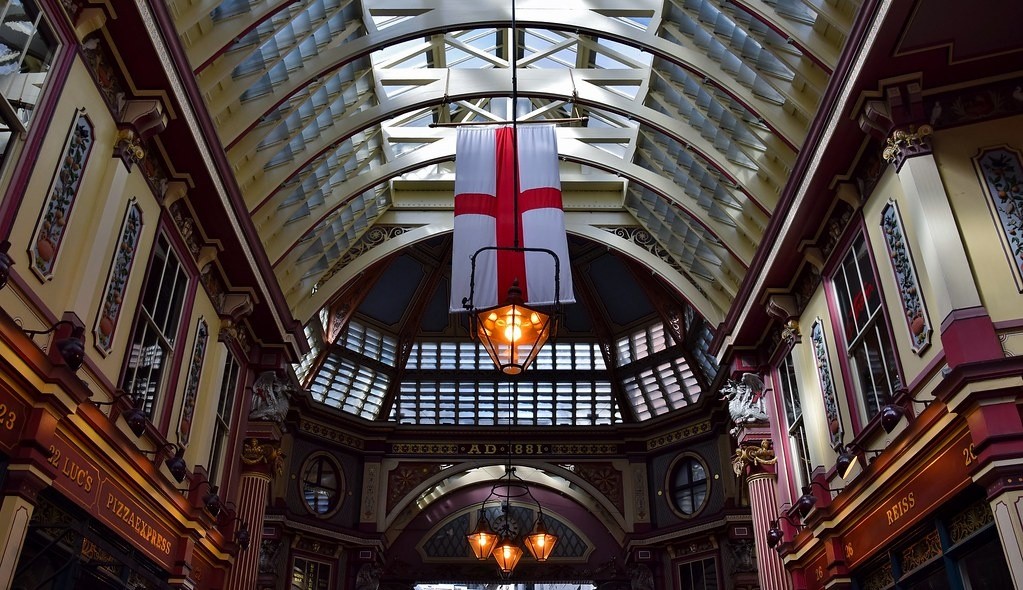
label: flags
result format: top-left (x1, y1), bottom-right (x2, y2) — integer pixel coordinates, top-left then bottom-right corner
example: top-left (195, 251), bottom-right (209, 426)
top-left (446, 124), bottom-right (575, 310)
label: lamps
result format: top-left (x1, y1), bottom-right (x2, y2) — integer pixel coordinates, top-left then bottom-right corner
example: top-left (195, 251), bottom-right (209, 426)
top-left (140, 443), bottom-right (188, 484)
top-left (837, 443), bottom-right (884, 479)
top-left (178, 481), bottom-right (220, 517)
top-left (93, 392), bottom-right (147, 438)
top-left (20, 320), bottom-right (85, 372)
top-left (799, 482), bottom-right (844, 518)
top-left (221, 518), bottom-right (252, 552)
top-left (766, 517), bottom-right (807, 549)
top-left (468, 346), bottom-right (558, 574)
top-left (462, 0), bottom-right (561, 375)
top-left (881, 390), bottom-right (935, 433)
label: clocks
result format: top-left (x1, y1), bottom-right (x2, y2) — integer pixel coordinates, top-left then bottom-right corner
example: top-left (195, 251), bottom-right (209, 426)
top-left (492, 514), bottom-right (520, 542)
